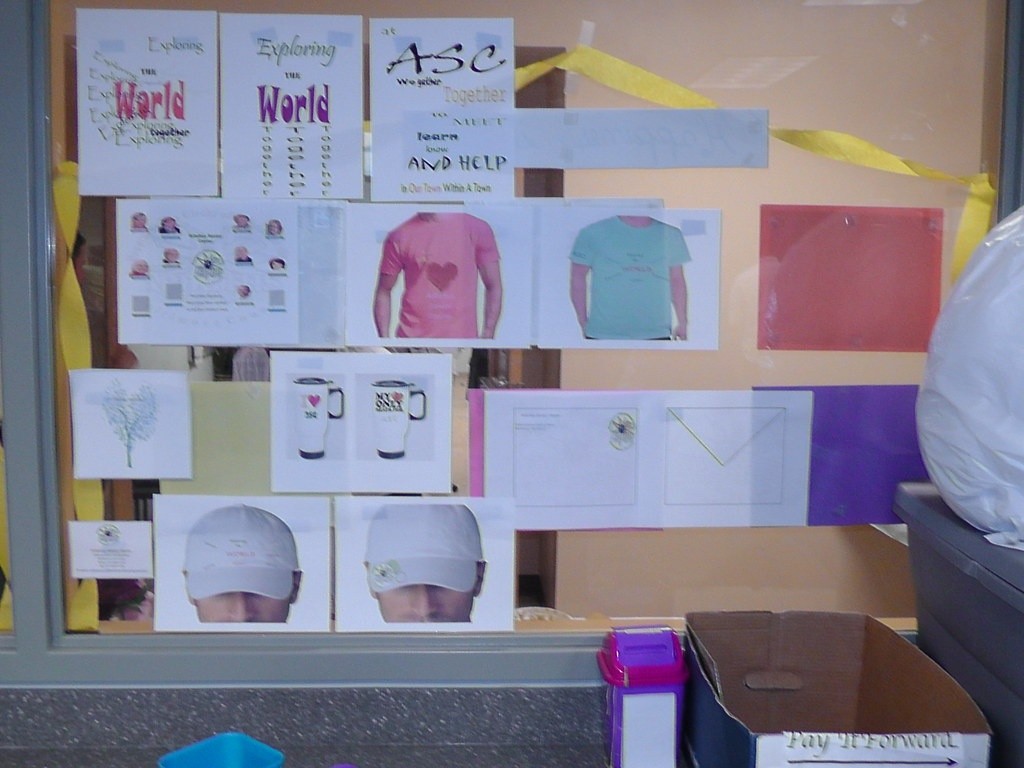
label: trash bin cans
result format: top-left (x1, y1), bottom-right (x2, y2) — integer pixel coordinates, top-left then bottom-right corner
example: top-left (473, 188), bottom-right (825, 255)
top-left (597, 624), bottom-right (689, 768)
top-left (892, 481), bottom-right (1024, 768)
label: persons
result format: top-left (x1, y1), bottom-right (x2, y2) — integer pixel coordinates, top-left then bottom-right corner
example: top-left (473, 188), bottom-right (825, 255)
top-left (566, 216), bottom-right (695, 340)
top-left (128, 209), bottom-right (290, 305)
top-left (180, 503), bottom-right (303, 624)
top-left (371, 213), bottom-right (504, 340)
top-left (362, 502), bottom-right (488, 624)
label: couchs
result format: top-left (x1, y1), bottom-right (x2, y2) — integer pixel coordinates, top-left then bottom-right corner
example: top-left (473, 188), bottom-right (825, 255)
top-left (894, 481), bottom-right (1024, 768)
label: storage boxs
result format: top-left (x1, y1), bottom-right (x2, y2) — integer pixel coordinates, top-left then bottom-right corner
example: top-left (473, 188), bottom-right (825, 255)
top-left (682, 609), bottom-right (993, 768)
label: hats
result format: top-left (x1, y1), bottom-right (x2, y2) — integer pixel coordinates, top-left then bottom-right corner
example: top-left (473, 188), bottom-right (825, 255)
top-left (363, 505), bottom-right (483, 594)
top-left (181, 503), bottom-right (299, 601)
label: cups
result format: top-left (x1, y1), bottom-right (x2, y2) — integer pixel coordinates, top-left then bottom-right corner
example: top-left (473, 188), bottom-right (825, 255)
top-left (291, 377), bottom-right (345, 460)
top-left (370, 380), bottom-right (429, 459)
